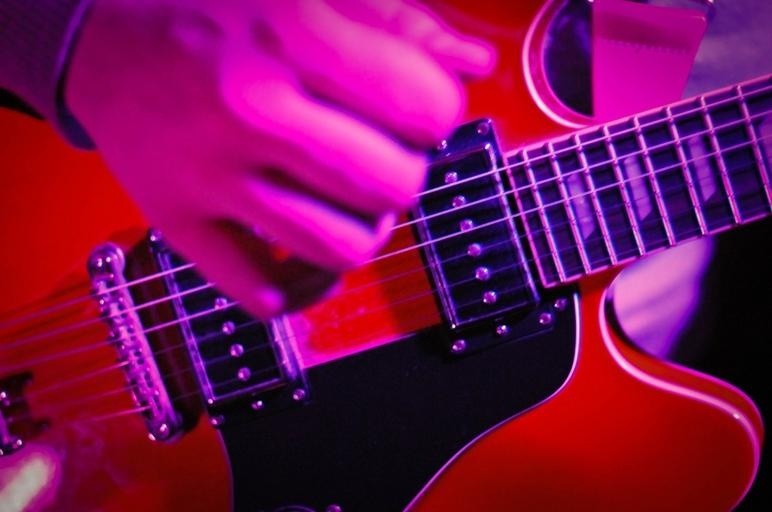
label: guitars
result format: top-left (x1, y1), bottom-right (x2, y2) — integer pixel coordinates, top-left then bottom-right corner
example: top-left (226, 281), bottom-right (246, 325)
top-left (0, 0), bottom-right (772, 509)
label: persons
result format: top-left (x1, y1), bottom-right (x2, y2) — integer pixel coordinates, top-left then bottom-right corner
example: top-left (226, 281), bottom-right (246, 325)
top-left (1, 0), bottom-right (498, 326)
